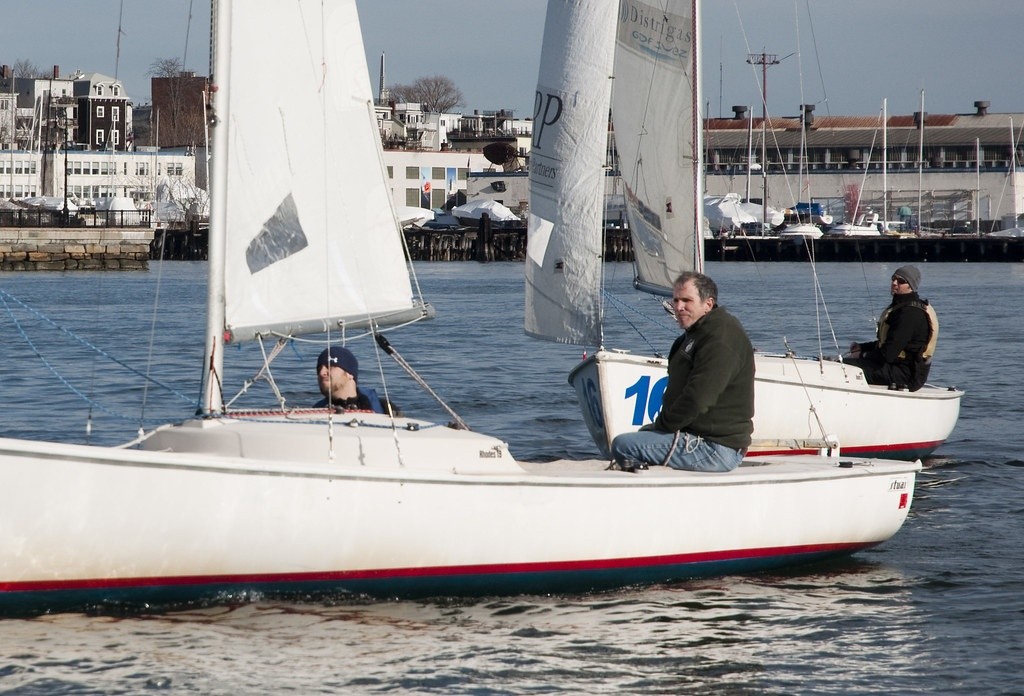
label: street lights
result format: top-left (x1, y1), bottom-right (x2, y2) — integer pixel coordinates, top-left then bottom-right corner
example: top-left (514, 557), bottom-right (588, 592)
top-left (50, 106), bottom-right (69, 228)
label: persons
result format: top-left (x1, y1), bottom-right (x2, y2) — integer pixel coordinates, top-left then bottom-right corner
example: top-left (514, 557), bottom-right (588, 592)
top-left (611, 272), bottom-right (755, 472)
top-left (838, 266), bottom-right (939, 392)
top-left (311, 347), bottom-right (400, 415)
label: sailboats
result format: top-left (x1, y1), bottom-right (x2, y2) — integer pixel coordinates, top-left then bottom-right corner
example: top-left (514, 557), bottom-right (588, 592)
top-left (520, 1), bottom-right (967, 460)
top-left (1, 0), bottom-right (933, 611)
top-left (697, 87), bottom-right (1024, 240)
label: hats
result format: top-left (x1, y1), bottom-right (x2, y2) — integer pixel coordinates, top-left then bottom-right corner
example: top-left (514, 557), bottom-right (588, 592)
top-left (317, 347), bottom-right (358, 382)
top-left (894, 266), bottom-right (922, 293)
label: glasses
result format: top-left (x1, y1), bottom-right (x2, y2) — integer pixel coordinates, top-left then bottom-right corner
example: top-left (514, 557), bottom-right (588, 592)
top-left (892, 275), bottom-right (909, 284)
